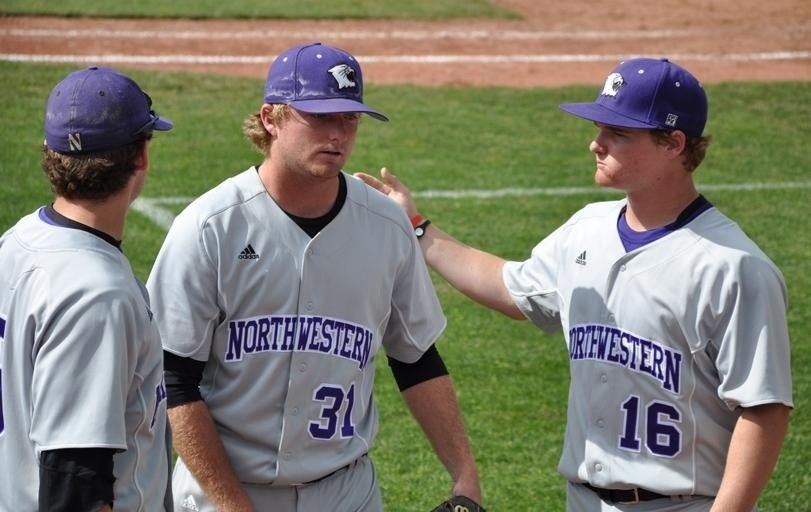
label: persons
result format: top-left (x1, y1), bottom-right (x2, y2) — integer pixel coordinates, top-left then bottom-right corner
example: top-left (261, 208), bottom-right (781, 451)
top-left (352, 57), bottom-right (795, 512)
top-left (144, 43), bottom-right (481, 512)
top-left (0, 65), bottom-right (176, 511)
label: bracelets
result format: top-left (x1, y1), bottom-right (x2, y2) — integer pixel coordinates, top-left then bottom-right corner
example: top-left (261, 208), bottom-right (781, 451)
top-left (414, 219), bottom-right (431, 240)
top-left (409, 213), bottom-right (424, 225)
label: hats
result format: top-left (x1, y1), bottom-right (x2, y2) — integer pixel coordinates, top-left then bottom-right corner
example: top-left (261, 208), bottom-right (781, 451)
top-left (262, 40), bottom-right (390, 124)
top-left (44, 65), bottom-right (173, 151)
top-left (560, 57), bottom-right (709, 138)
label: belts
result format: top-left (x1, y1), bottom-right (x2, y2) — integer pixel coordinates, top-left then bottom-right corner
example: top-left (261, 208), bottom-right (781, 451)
top-left (581, 480), bottom-right (695, 504)
